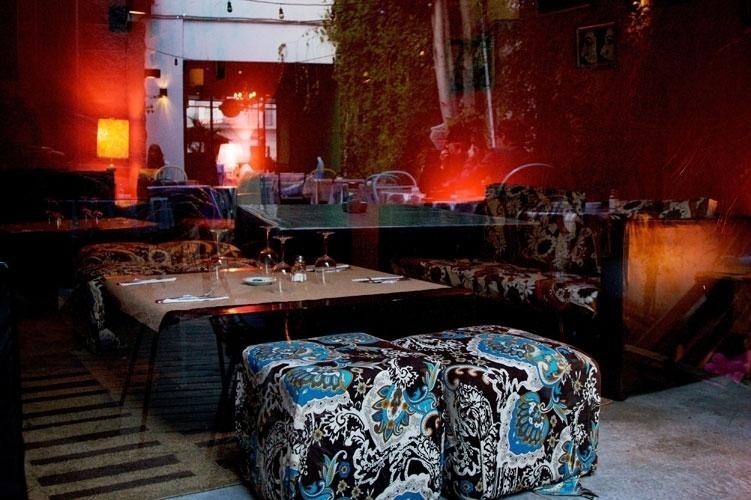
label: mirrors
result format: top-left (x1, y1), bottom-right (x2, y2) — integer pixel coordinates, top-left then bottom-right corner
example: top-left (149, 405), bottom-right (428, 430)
top-left (184, 61), bottom-right (338, 186)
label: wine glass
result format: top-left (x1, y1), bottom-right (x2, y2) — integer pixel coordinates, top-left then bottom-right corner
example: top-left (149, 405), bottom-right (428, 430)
top-left (210, 228), bottom-right (229, 298)
top-left (314, 231), bottom-right (336, 286)
top-left (257, 224), bottom-right (293, 295)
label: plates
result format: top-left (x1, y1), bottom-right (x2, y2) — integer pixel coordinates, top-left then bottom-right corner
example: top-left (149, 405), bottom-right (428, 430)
top-left (241, 276), bottom-right (275, 287)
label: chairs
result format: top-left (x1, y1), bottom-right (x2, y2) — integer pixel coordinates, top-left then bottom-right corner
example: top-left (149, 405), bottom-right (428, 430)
top-left (76, 165), bottom-right (418, 372)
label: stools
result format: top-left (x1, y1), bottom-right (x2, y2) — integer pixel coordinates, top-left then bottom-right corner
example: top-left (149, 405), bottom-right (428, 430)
top-left (391, 325), bottom-right (602, 500)
top-left (226, 332), bottom-right (446, 500)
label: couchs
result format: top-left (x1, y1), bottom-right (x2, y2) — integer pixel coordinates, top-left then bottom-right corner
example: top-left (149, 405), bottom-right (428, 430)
top-left (376, 201), bottom-right (751, 402)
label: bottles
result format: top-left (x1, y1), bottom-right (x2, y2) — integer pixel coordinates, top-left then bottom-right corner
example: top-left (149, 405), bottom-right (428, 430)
top-left (291, 254), bottom-right (309, 289)
top-left (607, 188), bottom-right (620, 212)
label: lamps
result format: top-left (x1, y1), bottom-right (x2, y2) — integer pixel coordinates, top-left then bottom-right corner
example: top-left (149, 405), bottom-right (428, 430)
top-left (227, 0), bottom-right (433, 19)
top-left (189, 68), bottom-right (204, 95)
top-left (96, 118), bottom-right (130, 169)
top-left (233, 81), bottom-right (257, 108)
top-left (144, 77), bottom-right (167, 98)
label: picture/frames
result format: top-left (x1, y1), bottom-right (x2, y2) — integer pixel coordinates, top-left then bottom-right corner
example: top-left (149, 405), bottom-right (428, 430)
top-left (534, 0), bottom-right (591, 18)
top-left (575, 21), bottom-right (617, 70)
top-left (450, 39), bottom-right (491, 91)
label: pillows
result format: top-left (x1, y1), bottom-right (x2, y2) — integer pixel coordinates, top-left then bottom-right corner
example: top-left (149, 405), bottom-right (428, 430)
top-left (483, 184), bottom-right (719, 276)
